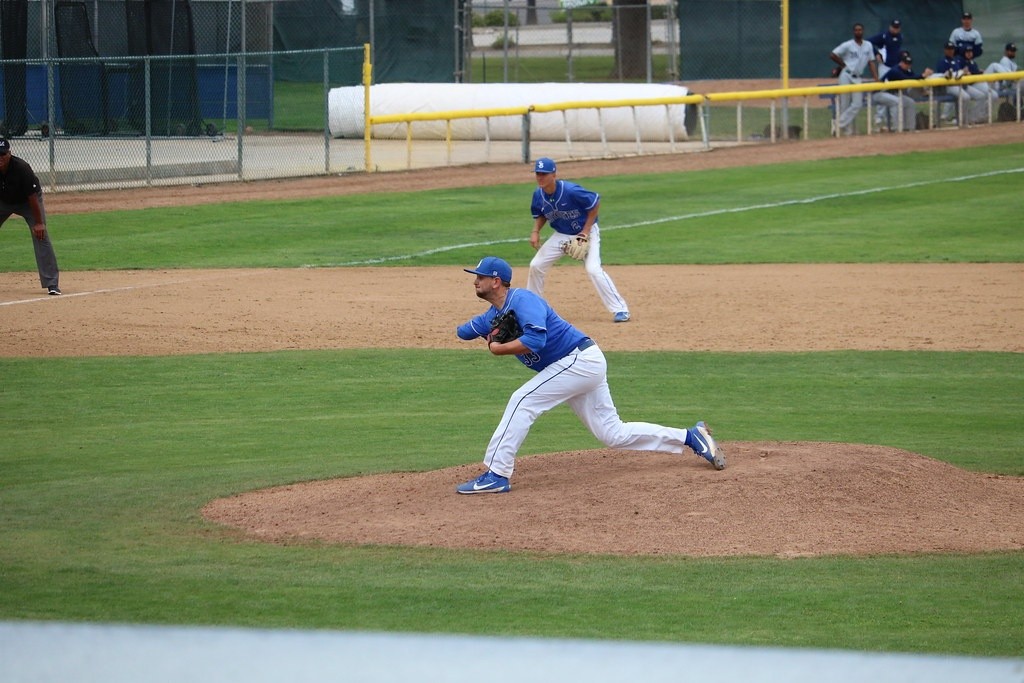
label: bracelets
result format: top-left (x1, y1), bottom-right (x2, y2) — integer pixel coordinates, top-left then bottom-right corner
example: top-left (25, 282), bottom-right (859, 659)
top-left (531, 231), bottom-right (539, 232)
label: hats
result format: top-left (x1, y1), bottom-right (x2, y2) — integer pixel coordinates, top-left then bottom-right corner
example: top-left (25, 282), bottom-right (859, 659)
top-left (0, 138), bottom-right (11, 148)
top-left (944, 41), bottom-right (954, 49)
top-left (531, 158), bottom-right (556, 174)
top-left (960, 12), bottom-right (972, 19)
top-left (463, 256), bottom-right (512, 283)
top-left (1005, 42), bottom-right (1018, 52)
top-left (890, 18), bottom-right (901, 28)
top-left (898, 49), bottom-right (913, 65)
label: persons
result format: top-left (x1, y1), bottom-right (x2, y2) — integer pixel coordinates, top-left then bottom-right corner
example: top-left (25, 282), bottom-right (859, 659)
top-left (871, 51), bottom-right (933, 133)
top-left (865, 17), bottom-right (903, 132)
top-left (526, 157), bottom-right (631, 322)
top-left (940, 12), bottom-right (983, 120)
top-left (455, 256), bottom-right (726, 495)
top-left (937, 41), bottom-right (999, 126)
top-left (830, 23), bottom-right (883, 138)
top-left (0, 137), bottom-right (62, 295)
top-left (1000, 43), bottom-right (1024, 89)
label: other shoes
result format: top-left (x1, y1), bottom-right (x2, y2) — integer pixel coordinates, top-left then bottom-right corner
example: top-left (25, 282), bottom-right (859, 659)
top-left (830, 119), bottom-right (837, 137)
top-left (47, 285), bottom-right (62, 295)
top-left (614, 311), bottom-right (631, 323)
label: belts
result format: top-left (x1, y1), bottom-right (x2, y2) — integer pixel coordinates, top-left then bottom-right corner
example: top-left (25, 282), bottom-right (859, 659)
top-left (846, 70), bottom-right (857, 79)
top-left (579, 339), bottom-right (595, 351)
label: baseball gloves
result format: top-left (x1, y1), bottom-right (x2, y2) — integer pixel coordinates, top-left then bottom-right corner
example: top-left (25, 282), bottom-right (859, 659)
top-left (486, 309), bottom-right (521, 350)
top-left (560, 232), bottom-right (590, 261)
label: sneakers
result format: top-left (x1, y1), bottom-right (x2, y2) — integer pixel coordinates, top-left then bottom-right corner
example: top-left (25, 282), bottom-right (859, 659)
top-left (456, 469), bottom-right (512, 494)
top-left (688, 421), bottom-right (726, 471)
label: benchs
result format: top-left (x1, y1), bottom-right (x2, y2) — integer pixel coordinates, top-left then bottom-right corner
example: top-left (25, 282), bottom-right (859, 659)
top-left (817, 74), bottom-right (1024, 134)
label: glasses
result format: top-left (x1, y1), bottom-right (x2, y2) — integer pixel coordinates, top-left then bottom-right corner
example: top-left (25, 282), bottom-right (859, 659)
top-left (0, 150), bottom-right (9, 156)
